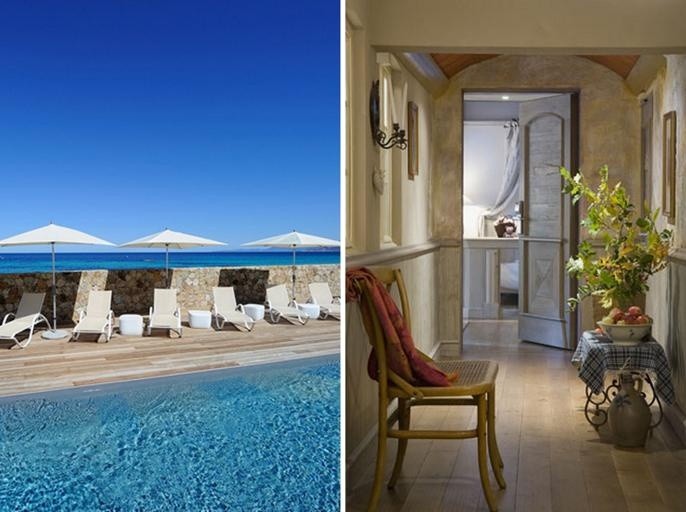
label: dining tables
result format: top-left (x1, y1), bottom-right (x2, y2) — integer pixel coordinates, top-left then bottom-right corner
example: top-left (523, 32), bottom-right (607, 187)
top-left (557, 167), bottom-right (677, 329)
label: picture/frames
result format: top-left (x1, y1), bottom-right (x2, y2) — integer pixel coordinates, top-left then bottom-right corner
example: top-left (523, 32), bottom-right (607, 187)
top-left (120, 314), bottom-right (145, 338)
top-left (581, 331), bottom-right (664, 431)
top-left (188, 309), bottom-right (212, 329)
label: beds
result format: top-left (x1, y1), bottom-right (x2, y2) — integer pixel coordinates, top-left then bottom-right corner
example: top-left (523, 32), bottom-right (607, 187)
top-left (241, 230), bottom-right (340, 307)
top-left (119, 229), bottom-right (228, 288)
top-left (0, 220), bottom-right (116, 329)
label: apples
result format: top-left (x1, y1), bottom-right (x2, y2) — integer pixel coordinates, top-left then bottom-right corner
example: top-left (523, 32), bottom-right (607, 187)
top-left (388, 76), bottom-right (408, 129)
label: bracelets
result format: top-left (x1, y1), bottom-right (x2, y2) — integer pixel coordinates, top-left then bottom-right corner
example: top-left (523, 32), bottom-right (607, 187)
top-left (596, 320), bottom-right (651, 347)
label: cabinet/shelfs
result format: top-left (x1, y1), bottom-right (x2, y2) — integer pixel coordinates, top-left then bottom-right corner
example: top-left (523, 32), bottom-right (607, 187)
top-left (609, 371), bottom-right (652, 448)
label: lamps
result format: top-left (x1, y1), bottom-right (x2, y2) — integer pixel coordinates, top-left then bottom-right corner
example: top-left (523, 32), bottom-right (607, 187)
top-left (147, 288), bottom-right (183, 340)
top-left (212, 282), bottom-right (342, 334)
top-left (351, 267), bottom-right (505, 512)
top-left (1, 291), bottom-right (52, 349)
top-left (71, 290), bottom-right (116, 344)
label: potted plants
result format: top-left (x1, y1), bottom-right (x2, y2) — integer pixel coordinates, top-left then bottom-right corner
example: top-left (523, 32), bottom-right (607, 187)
top-left (408, 100), bottom-right (419, 175)
top-left (660, 110), bottom-right (676, 217)
